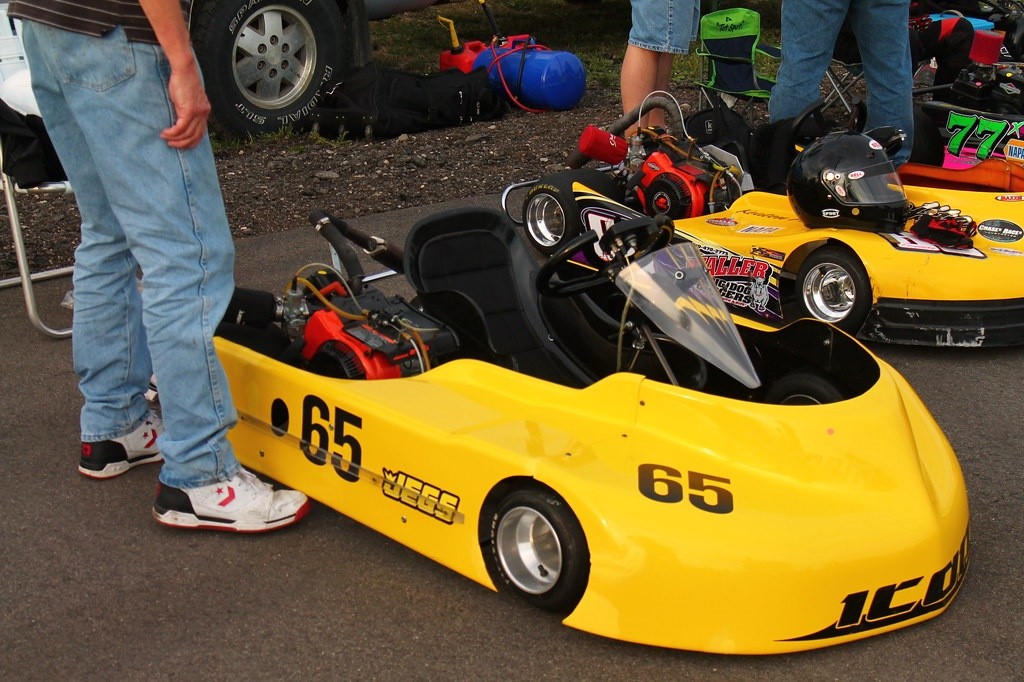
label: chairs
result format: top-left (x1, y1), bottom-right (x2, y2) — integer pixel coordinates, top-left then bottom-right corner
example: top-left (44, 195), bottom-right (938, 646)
top-left (686, 108), bottom-right (752, 170)
top-left (0, 3), bottom-right (72, 340)
top-left (403, 205), bottom-right (622, 389)
top-left (694, 7), bottom-right (781, 124)
top-left (821, 25), bottom-right (864, 117)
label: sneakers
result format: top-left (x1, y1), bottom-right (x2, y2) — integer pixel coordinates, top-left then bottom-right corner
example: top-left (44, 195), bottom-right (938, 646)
top-left (79, 410), bottom-right (163, 479)
top-left (152, 466), bottom-right (312, 532)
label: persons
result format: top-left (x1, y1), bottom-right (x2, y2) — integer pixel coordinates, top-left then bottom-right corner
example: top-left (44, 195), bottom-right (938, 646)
top-left (2, 1), bottom-right (314, 535)
top-left (834, 16), bottom-right (975, 101)
top-left (619, 1), bottom-right (703, 159)
top-left (767, 0), bottom-right (915, 170)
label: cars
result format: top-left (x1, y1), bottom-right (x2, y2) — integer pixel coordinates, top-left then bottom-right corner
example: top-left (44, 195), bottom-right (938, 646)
top-left (190, 0), bottom-right (501, 141)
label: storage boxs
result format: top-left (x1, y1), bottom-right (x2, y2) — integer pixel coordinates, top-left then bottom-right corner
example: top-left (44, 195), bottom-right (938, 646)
top-left (930, 13), bottom-right (995, 31)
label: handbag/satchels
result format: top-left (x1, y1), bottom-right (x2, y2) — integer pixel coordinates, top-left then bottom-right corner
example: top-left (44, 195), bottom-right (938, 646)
top-left (322, 69), bottom-right (508, 143)
top-left (683, 105), bottom-right (845, 196)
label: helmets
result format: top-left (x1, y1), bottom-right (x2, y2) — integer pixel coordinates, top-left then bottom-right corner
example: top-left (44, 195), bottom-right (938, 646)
top-left (786, 135), bottom-right (910, 234)
